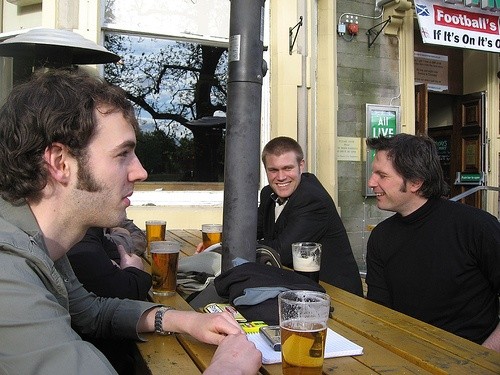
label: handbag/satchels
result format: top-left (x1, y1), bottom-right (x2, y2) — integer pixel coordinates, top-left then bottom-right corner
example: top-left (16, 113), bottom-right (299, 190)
top-left (178, 244), bottom-right (282, 283)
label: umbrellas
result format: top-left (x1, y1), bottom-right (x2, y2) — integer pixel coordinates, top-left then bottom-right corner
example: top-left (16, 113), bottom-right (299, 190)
top-left (0, 28), bottom-right (120, 65)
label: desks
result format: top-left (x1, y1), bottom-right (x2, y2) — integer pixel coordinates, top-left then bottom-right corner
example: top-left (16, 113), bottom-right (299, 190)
top-left (135, 228), bottom-right (500, 375)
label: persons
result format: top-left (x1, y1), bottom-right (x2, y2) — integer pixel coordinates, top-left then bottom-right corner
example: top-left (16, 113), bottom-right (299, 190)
top-left (194, 136), bottom-right (364, 298)
top-left (67, 217), bottom-right (153, 375)
top-left (366, 134), bottom-right (500, 353)
top-left (0, 69), bottom-right (262, 375)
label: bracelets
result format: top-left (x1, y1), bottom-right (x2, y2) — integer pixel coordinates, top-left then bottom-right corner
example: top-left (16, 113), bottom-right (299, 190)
top-left (155, 306), bottom-right (176, 335)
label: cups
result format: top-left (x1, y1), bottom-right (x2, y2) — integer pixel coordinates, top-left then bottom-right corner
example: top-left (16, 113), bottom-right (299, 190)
top-left (146, 220), bottom-right (167, 257)
top-left (149, 241), bottom-right (180, 296)
top-left (292, 242), bottom-right (321, 284)
top-left (202, 224), bottom-right (223, 251)
top-left (278, 290), bottom-right (330, 375)
